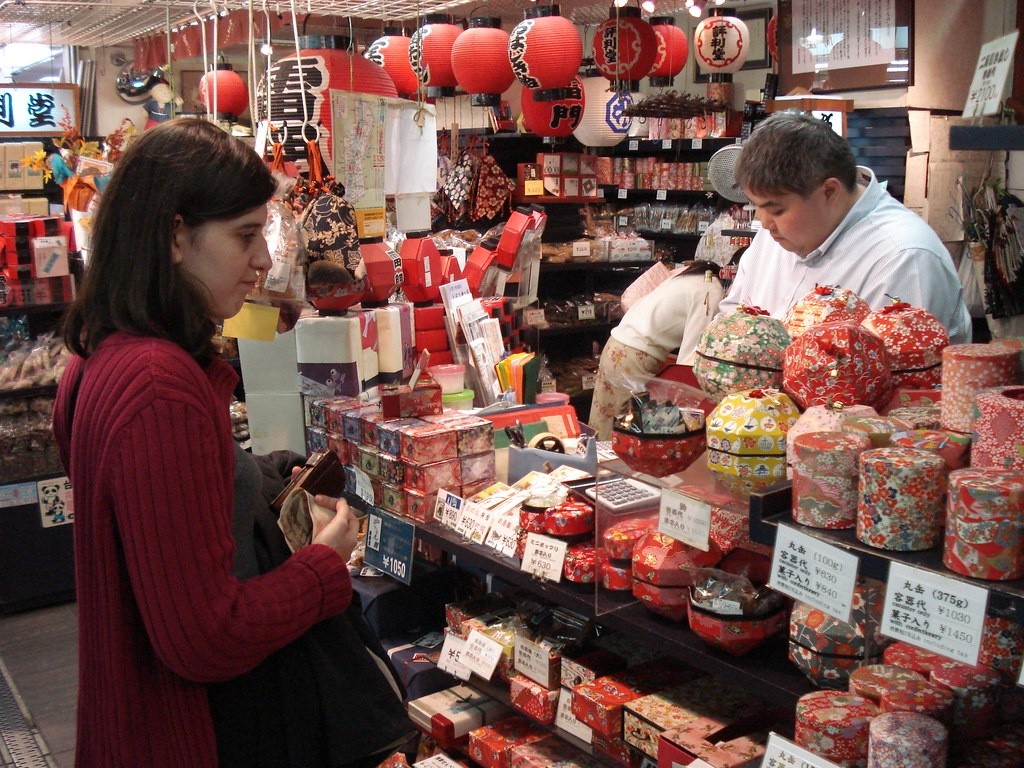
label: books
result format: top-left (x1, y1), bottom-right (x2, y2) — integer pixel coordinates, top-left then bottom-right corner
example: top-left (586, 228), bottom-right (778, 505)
top-left (438, 277), bottom-right (543, 409)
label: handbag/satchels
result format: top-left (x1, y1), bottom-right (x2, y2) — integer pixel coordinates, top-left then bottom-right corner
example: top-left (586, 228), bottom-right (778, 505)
top-left (203, 509), bottom-right (422, 768)
top-left (245, 140), bottom-right (373, 336)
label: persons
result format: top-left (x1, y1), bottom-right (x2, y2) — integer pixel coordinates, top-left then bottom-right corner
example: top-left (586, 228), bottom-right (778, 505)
top-left (693, 193), bottom-right (748, 267)
top-left (718, 109), bottom-right (972, 348)
top-left (51, 117), bottom-right (360, 767)
top-left (588, 245), bottom-right (750, 442)
top-left (142, 77), bottom-right (185, 133)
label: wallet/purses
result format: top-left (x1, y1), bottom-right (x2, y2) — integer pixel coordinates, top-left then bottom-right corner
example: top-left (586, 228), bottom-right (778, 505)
top-left (270, 446), bottom-right (347, 517)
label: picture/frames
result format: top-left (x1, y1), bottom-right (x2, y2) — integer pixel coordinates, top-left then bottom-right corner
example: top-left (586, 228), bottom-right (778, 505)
top-left (180, 69), bottom-right (251, 121)
top-left (736, 8), bottom-right (772, 71)
top-left (777, 0), bottom-right (914, 95)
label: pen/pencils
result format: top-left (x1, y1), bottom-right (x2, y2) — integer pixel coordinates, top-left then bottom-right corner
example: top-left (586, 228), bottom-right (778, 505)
top-left (504, 419), bottom-right (527, 448)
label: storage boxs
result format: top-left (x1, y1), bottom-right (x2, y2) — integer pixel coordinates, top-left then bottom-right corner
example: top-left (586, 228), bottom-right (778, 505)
top-left (0, 137), bottom-right (83, 308)
top-left (352, 556), bottom-right (794, 768)
top-left (303, 105), bottom-right (743, 524)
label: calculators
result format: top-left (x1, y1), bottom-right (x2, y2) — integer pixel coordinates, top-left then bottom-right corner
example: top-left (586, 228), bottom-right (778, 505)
top-left (585, 477), bottom-right (662, 512)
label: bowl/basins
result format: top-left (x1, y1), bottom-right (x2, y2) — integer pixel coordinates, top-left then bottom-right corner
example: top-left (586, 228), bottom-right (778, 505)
top-left (441, 389), bottom-right (474, 410)
top-left (429, 364), bottom-right (465, 395)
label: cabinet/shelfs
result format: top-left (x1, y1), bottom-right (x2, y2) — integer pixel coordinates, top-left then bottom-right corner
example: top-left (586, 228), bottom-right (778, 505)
top-left (600, 136), bottom-right (736, 242)
top-left (338, 488), bottom-right (1024, 768)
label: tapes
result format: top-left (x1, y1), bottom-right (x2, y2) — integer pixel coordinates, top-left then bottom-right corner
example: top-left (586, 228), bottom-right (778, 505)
top-left (527, 432), bottom-right (565, 454)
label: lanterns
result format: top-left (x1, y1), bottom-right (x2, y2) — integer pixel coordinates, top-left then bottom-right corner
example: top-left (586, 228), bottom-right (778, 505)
top-left (198, 63), bottom-right (249, 124)
top-left (362, 4), bottom-right (688, 143)
top-left (253, 36), bottom-right (400, 191)
top-left (694, 7), bottom-right (751, 83)
